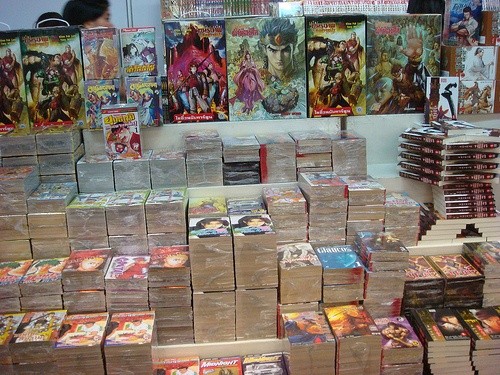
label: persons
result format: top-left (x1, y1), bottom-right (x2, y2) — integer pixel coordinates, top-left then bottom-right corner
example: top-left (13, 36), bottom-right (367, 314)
top-left (35, 0), bottom-right (112, 28)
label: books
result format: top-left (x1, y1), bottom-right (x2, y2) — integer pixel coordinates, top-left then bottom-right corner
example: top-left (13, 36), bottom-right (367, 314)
top-left (162, 0), bottom-right (500, 124)
top-left (0, 25), bottom-right (163, 130)
top-left (0, 120), bottom-right (500, 375)
